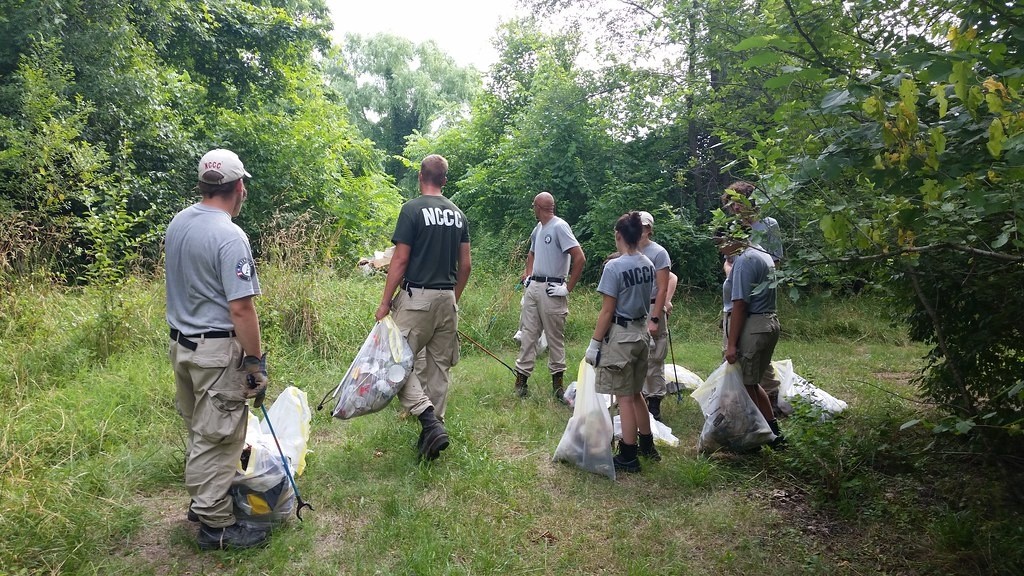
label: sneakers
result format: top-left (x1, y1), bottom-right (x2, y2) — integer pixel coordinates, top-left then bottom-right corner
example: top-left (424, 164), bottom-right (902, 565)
top-left (188, 499), bottom-right (199, 522)
top-left (197, 522), bottom-right (268, 551)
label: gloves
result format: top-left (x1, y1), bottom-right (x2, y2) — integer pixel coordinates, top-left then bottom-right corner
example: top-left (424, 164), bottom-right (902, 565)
top-left (546, 282), bottom-right (570, 296)
top-left (239, 353), bottom-right (267, 408)
top-left (585, 338), bottom-right (602, 367)
top-left (524, 276), bottom-right (531, 287)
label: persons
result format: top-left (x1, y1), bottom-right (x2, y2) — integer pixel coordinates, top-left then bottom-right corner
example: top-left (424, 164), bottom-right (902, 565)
top-left (515, 192), bottom-right (586, 402)
top-left (375, 154), bottom-right (471, 463)
top-left (166, 150), bottom-right (267, 549)
top-left (665, 271), bottom-right (677, 315)
top-left (639, 211), bottom-right (672, 423)
top-left (585, 212), bottom-right (661, 473)
top-left (722, 182), bottom-right (793, 418)
top-left (716, 216), bottom-right (788, 451)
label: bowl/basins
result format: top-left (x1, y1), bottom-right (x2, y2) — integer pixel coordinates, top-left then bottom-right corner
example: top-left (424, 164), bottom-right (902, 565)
top-left (388, 365), bottom-right (405, 383)
top-left (332, 400), bottom-right (355, 419)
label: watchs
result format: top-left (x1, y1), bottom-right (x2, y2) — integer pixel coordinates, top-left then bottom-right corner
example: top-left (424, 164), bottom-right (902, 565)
top-left (651, 317), bottom-right (659, 323)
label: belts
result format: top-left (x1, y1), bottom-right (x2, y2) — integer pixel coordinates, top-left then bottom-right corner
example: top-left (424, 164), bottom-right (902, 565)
top-left (651, 299), bottom-right (655, 304)
top-left (169, 329), bottom-right (236, 351)
top-left (611, 316), bottom-right (644, 327)
top-left (401, 281), bottom-right (454, 298)
top-left (531, 276), bottom-right (561, 283)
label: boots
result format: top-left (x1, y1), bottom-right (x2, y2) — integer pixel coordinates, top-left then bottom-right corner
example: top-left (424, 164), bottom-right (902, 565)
top-left (646, 396), bottom-right (662, 421)
top-left (418, 406), bottom-right (450, 462)
top-left (638, 431), bottom-right (661, 461)
top-left (752, 419), bottom-right (788, 453)
top-left (552, 372), bottom-right (567, 405)
top-left (613, 439), bottom-right (641, 472)
top-left (513, 372), bottom-right (527, 399)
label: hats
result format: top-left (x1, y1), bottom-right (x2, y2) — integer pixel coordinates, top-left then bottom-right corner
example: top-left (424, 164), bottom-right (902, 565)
top-left (639, 211), bottom-right (654, 228)
top-left (198, 149), bottom-right (252, 185)
